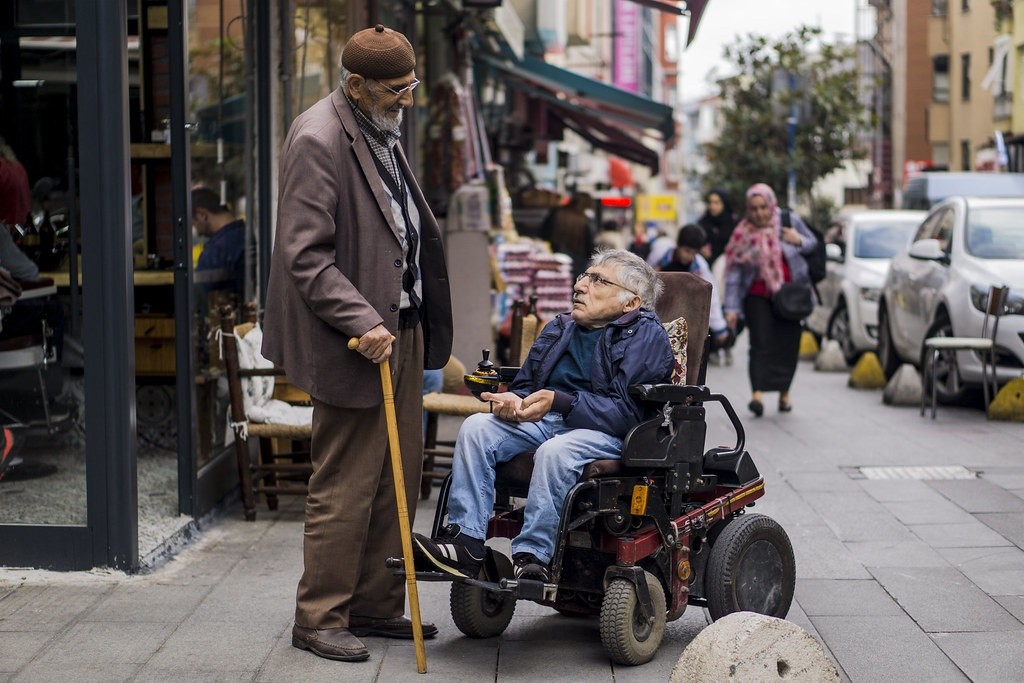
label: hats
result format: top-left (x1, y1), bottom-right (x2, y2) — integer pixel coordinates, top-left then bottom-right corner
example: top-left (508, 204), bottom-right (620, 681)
top-left (342, 25), bottom-right (415, 79)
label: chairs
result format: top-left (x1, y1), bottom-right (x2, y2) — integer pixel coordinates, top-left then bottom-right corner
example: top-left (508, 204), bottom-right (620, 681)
top-left (422, 295), bottom-right (540, 497)
top-left (0, 277), bottom-right (75, 481)
top-left (220, 298), bottom-right (315, 522)
top-left (921, 285), bottom-right (1010, 420)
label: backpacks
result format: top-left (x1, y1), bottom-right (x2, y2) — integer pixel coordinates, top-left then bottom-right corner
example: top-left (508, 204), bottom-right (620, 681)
top-left (780, 208), bottom-right (826, 284)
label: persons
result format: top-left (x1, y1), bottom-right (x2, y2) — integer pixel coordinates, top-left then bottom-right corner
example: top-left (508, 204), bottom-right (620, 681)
top-left (259, 24), bottom-right (455, 660)
top-left (654, 222), bottom-right (729, 390)
top-left (725, 184), bottom-right (817, 414)
top-left (628, 220), bottom-right (653, 263)
top-left (696, 189), bottom-right (743, 366)
top-left (0, 134), bottom-right (73, 430)
top-left (540, 190), bottom-right (594, 282)
top-left (591, 219), bottom-right (626, 251)
top-left (190, 186), bottom-right (264, 402)
top-left (411, 244), bottom-right (675, 589)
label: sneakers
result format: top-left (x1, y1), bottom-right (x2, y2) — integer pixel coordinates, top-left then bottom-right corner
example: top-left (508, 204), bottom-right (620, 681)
top-left (0, 429), bottom-right (29, 481)
top-left (512, 552), bottom-right (550, 583)
top-left (411, 523), bottom-right (483, 581)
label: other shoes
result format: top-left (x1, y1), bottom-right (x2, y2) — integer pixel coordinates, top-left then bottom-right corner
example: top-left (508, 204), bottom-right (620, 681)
top-left (748, 400), bottom-right (763, 416)
top-left (779, 401), bottom-right (791, 411)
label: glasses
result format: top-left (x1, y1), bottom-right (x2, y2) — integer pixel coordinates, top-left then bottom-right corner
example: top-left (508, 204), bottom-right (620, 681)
top-left (373, 78), bottom-right (420, 99)
top-left (576, 273), bottom-right (637, 296)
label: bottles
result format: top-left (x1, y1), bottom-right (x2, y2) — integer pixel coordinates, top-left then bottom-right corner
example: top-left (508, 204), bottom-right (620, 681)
top-left (511, 295), bottom-right (540, 367)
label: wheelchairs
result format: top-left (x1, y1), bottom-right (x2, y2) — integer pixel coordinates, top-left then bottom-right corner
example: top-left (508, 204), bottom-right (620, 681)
top-left (0, 266), bottom-right (79, 478)
top-left (381, 273), bottom-right (796, 668)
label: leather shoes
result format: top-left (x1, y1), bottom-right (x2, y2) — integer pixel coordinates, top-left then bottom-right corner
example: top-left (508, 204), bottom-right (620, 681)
top-left (349, 614), bottom-right (438, 638)
top-left (292, 622), bottom-right (370, 661)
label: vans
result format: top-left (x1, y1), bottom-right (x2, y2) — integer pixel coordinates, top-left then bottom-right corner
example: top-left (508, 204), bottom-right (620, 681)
top-left (898, 172), bottom-right (1024, 212)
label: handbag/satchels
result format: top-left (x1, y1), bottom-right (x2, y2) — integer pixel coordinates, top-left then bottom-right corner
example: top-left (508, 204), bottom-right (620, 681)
top-left (773, 285), bottom-right (813, 320)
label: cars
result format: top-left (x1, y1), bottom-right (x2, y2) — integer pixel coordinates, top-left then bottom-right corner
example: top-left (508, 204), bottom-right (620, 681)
top-left (802, 208), bottom-right (929, 365)
top-left (876, 196), bottom-right (1024, 407)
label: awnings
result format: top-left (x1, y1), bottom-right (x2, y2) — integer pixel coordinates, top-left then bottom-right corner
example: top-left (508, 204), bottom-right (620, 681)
top-left (518, 82), bottom-right (660, 178)
top-left (471, 36), bottom-right (677, 150)
